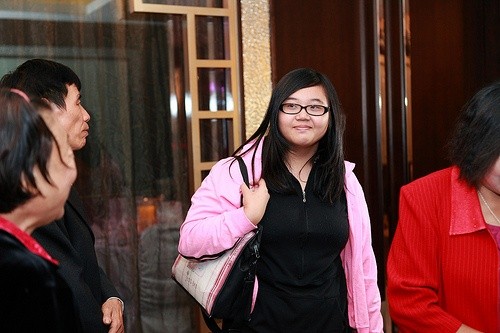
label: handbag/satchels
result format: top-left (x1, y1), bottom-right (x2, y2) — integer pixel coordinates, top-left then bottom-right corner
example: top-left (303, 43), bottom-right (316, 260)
top-left (171, 157), bottom-right (262, 333)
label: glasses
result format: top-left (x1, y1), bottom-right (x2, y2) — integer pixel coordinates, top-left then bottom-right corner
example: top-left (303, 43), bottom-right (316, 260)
top-left (279, 103), bottom-right (331, 116)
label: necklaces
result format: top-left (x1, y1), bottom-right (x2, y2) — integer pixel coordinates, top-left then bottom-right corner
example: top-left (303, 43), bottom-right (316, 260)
top-left (477, 189), bottom-right (500, 224)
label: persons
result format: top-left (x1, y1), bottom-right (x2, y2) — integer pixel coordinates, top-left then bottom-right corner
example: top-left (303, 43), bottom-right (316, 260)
top-left (386, 82), bottom-right (500, 333)
top-left (0, 59), bottom-right (129, 333)
top-left (177, 68), bottom-right (383, 333)
